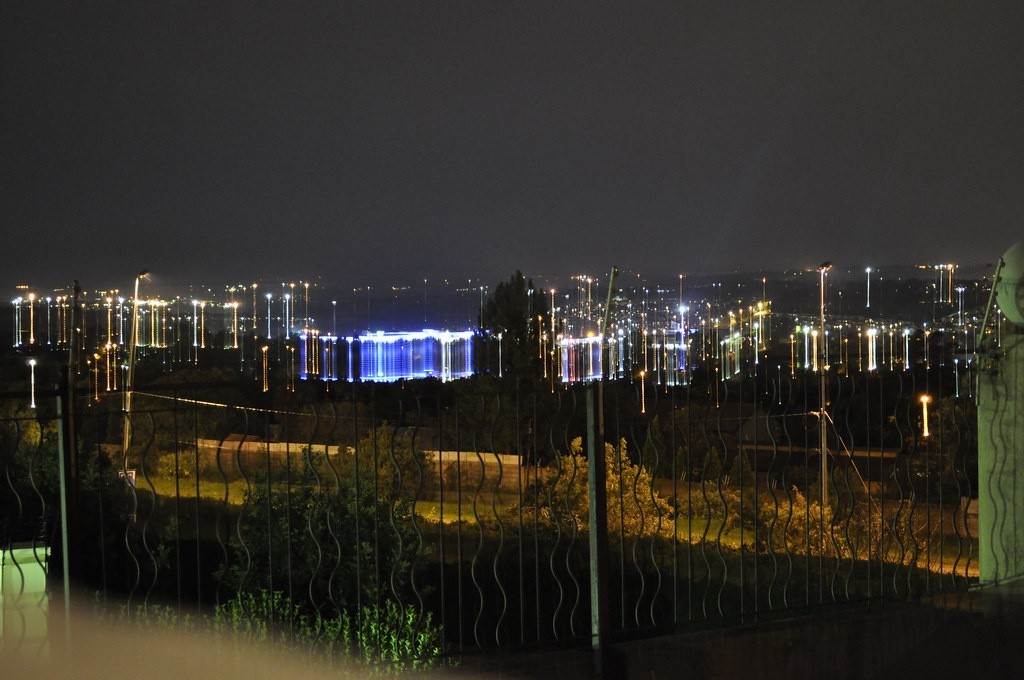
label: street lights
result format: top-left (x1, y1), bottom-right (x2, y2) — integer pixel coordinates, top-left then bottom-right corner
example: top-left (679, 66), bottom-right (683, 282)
top-left (548, 288), bottom-right (558, 350)
top-left (676, 274), bottom-right (688, 303)
top-left (919, 394), bottom-right (932, 436)
top-left (813, 262), bottom-right (833, 330)
top-left (864, 263), bottom-right (875, 310)
top-left (587, 278), bottom-right (594, 329)
top-left (13, 276), bottom-right (537, 398)
top-left (537, 314), bottom-right (543, 359)
top-left (26, 359), bottom-right (37, 409)
top-left (547, 258), bottom-right (1006, 403)
top-left (759, 275), bottom-right (769, 310)
top-left (496, 333), bottom-right (503, 377)
top-left (121, 268), bottom-right (152, 473)
